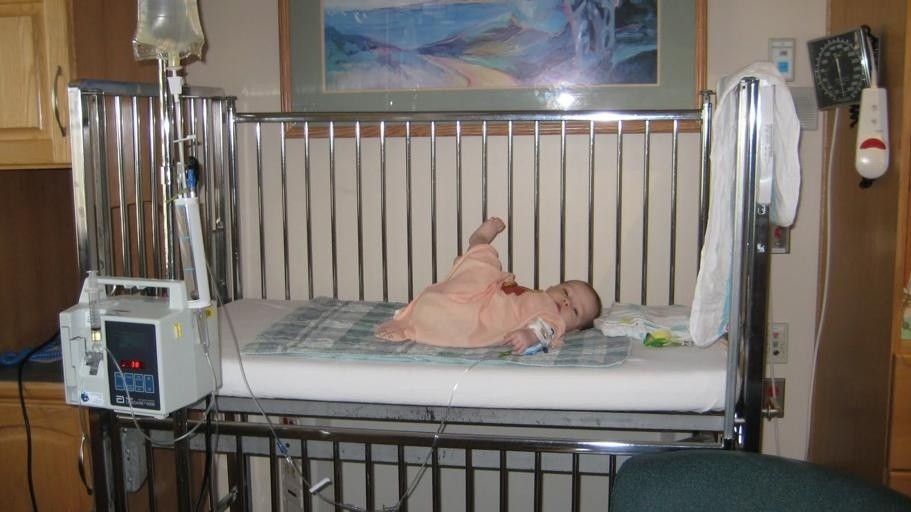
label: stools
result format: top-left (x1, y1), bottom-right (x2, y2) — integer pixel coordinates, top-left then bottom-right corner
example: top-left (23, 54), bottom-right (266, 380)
top-left (613, 448), bottom-right (910, 512)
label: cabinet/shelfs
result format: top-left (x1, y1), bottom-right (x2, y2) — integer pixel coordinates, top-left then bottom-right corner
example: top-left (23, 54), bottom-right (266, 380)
top-left (0, 3), bottom-right (158, 169)
top-left (2, 378), bottom-right (209, 511)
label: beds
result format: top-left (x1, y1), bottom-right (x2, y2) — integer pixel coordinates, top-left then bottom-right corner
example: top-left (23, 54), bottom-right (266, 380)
top-left (68, 81), bottom-right (770, 511)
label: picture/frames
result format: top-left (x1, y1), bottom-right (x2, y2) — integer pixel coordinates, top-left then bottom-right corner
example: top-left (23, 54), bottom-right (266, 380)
top-left (277, 0), bottom-right (711, 136)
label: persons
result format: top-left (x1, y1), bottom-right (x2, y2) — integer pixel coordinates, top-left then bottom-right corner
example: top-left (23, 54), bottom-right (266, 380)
top-left (375, 215), bottom-right (604, 359)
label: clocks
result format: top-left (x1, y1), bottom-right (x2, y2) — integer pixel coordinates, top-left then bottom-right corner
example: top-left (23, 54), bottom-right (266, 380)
top-left (808, 26), bottom-right (878, 113)
